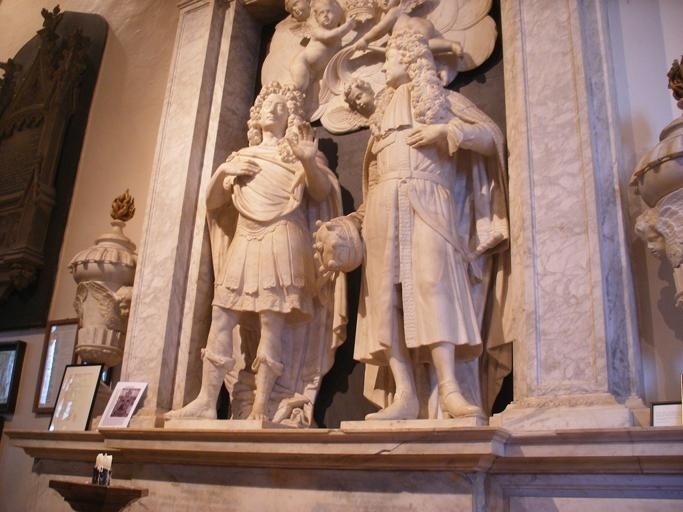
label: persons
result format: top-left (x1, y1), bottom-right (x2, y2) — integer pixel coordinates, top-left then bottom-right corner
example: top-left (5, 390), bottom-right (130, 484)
top-left (291, 1), bottom-right (356, 89)
top-left (632, 208), bottom-right (666, 260)
top-left (160, 79), bottom-right (347, 424)
top-left (350, 0), bottom-right (463, 59)
top-left (113, 285), bottom-right (134, 318)
top-left (347, 29), bottom-right (511, 420)
top-left (344, 78), bottom-right (374, 114)
top-left (283, 0), bottom-right (312, 23)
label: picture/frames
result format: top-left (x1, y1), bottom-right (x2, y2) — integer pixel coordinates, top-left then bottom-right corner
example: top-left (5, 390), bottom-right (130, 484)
top-left (47, 362), bottom-right (149, 432)
top-left (0, 316), bottom-right (111, 416)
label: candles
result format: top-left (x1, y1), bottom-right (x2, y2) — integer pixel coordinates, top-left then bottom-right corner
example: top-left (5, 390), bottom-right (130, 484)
top-left (95, 452), bottom-right (114, 471)
